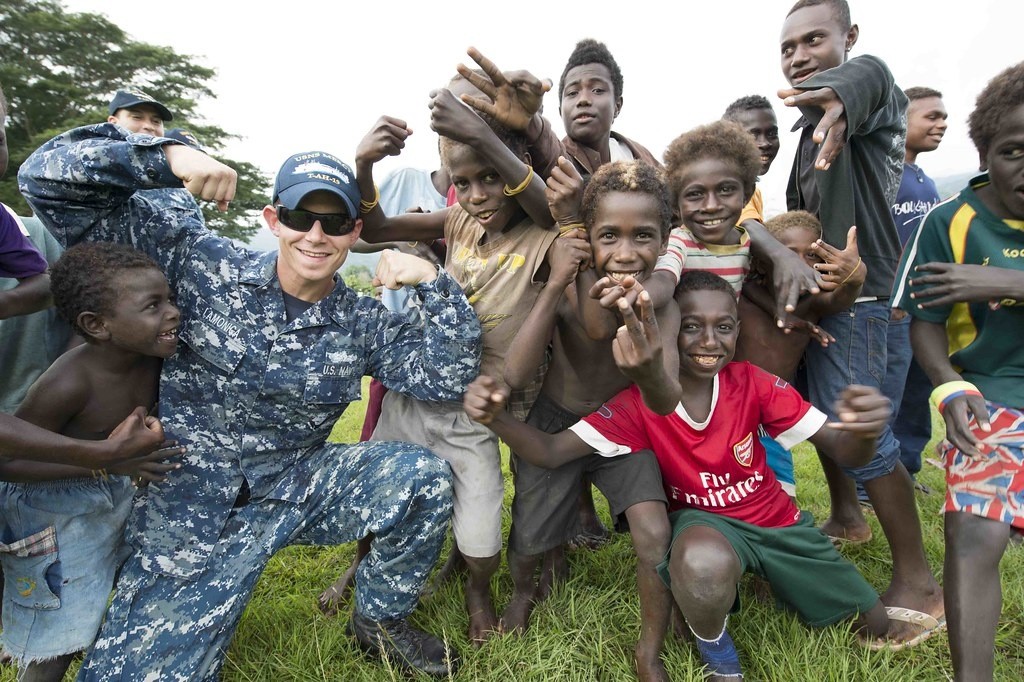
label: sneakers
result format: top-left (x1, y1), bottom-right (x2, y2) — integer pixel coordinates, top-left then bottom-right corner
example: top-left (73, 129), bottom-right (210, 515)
top-left (346, 608), bottom-right (464, 680)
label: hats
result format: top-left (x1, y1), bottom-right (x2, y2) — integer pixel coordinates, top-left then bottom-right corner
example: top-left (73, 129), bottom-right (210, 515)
top-left (109, 87), bottom-right (173, 121)
top-left (164, 127), bottom-right (208, 155)
top-left (272, 151), bottom-right (361, 219)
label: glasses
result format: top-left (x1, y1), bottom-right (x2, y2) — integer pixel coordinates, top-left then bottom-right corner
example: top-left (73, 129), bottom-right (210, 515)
top-left (275, 204), bottom-right (355, 236)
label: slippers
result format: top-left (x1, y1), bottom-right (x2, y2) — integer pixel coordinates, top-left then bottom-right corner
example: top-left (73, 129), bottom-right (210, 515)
top-left (827, 532), bottom-right (872, 550)
top-left (856, 607), bottom-right (948, 652)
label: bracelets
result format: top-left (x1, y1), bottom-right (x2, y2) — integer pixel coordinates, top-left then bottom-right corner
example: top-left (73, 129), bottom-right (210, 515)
top-left (425, 239), bottom-right (435, 247)
top-left (559, 228), bottom-right (586, 238)
top-left (503, 164), bottom-right (534, 194)
top-left (504, 170), bottom-right (533, 196)
top-left (360, 184), bottom-right (378, 204)
top-left (361, 190), bottom-right (380, 208)
top-left (839, 256), bottom-right (862, 286)
top-left (360, 208), bottom-right (371, 212)
top-left (559, 222), bottom-right (586, 232)
top-left (361, 211), bottom-right (368, 214)
top-left (929, 380), bottom-right (984, 419)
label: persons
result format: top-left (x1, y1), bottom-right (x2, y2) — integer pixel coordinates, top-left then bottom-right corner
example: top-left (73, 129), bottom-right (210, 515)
top-left (0, 82), bottom-right (485, 682)
top-left (317, 0), bottom-right (949, 682)
top-left (889, 65), bottom-right (1024, 681)
